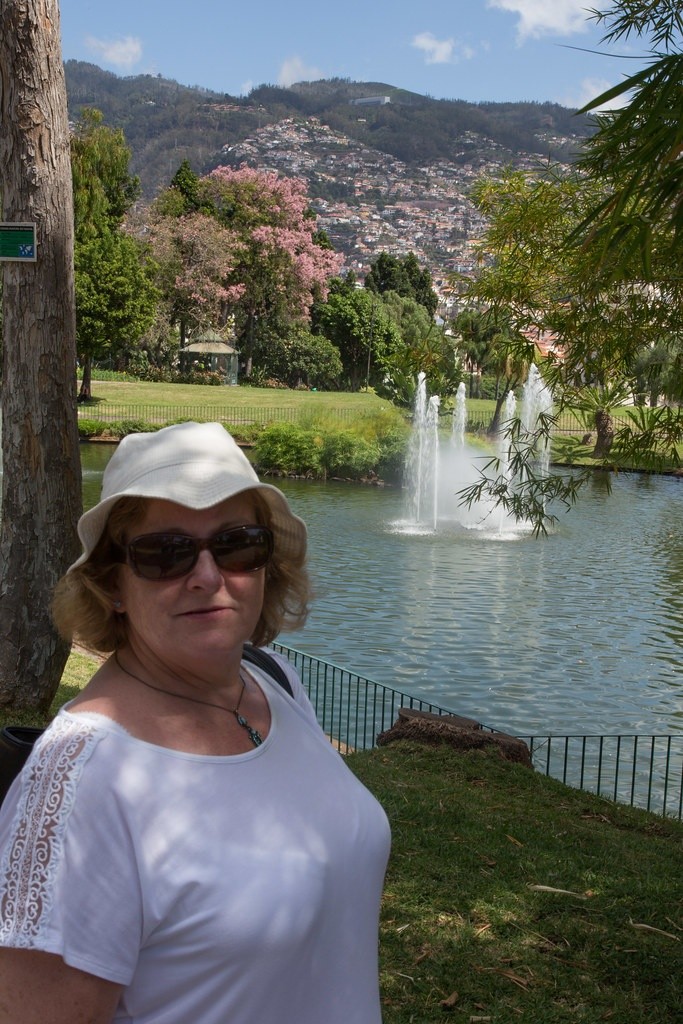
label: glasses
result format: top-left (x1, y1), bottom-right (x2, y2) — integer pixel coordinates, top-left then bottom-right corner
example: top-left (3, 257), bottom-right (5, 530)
top-left (108, 525), bottom-right (273, 582)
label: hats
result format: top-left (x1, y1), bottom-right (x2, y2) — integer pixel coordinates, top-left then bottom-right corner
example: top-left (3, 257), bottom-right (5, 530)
top-left (65, 420), bottom-right (308, 595)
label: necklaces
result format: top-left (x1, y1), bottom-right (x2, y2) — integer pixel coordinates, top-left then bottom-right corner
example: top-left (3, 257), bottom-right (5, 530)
top-left (114, 652), bottom-right (264, 748)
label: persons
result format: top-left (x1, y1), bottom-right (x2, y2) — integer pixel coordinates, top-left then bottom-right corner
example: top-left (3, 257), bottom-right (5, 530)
top-left (0, 421), bottom-right (392, 1022)
